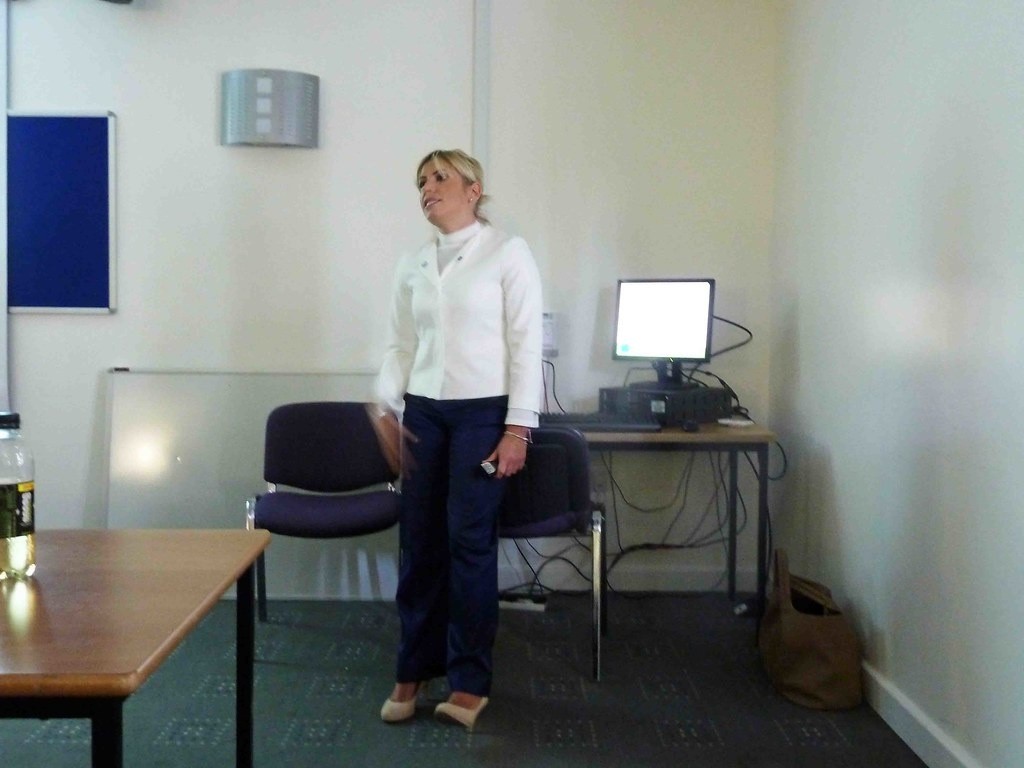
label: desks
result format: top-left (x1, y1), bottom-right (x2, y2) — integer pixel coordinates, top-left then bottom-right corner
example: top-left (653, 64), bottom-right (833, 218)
top-left (0, 527), bottom-right (270, 768)
top-left (582, 415), bottom-right (775, 649)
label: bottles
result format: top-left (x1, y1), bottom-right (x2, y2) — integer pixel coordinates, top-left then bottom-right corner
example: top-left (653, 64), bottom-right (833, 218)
top-left (0, 412), bottom-right (36, 582)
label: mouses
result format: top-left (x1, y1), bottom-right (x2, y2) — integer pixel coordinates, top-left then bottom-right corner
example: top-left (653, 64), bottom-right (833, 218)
top-left (680, 419), bottom-right (700, 432)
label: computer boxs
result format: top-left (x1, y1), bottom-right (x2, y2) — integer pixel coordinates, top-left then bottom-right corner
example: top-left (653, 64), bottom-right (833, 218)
top-left (599, 386), bottom-right (730, 429)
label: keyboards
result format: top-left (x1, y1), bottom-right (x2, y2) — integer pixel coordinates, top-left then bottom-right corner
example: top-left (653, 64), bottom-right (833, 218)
top-left (538, 410), bottom-right (662, 434)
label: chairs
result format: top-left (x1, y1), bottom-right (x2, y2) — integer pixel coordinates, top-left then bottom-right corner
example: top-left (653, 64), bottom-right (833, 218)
top-left (245, 401), bottom-right (405, 622)
top-left (495, 424), bottom-right (610, 685)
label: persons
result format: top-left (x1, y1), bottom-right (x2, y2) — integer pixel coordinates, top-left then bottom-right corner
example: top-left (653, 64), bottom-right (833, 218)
top-left (366, 149), bottom-right (545, 729)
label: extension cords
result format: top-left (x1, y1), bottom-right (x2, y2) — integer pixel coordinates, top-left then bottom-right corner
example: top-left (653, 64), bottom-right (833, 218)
top-left (499, 598), bottom-right (549, 613)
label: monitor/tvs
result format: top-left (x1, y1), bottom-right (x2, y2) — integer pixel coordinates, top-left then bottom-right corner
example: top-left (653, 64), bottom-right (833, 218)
top-left (613, 278), bottom-right (716, 392)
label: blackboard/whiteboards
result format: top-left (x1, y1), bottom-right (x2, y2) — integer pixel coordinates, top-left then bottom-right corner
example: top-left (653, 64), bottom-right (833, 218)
top-left (99, 366), bottom-right (400, 601)
top-left (6, 110), bottom-right (117, 314)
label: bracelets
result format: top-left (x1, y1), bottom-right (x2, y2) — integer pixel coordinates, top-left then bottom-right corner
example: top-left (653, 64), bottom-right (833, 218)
top-left (505, 431), bottom-right (529, 443)
